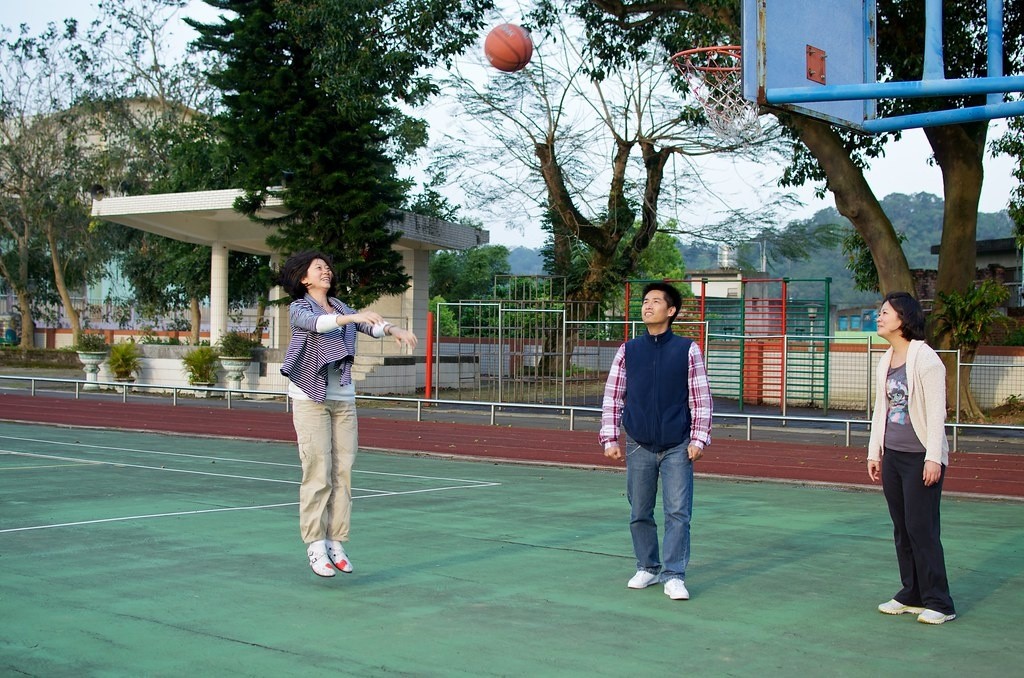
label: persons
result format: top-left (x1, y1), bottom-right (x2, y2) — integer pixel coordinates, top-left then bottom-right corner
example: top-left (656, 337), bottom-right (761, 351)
top-left (867, 293), bottom-right (956, 624)
top-left (280, 252), bottom-right (418, 577)
top-left (597, 282), bottom-right (714, 600)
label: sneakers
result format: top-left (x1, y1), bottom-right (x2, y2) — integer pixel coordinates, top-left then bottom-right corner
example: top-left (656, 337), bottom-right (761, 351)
top-left (628, 571), bottom-right (662, 588)
top-left (664, 578), bottom-right (690, 599)
top-left (917, 609), bottom-right (956, 624)
top-left (878, 598), bottom-right (926, 614)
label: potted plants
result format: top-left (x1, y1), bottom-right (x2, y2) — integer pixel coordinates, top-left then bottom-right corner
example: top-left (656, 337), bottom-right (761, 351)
top-left (76, 328), bottom-right (112, 392)
top-left (103, 336), bottom-right (145, 394)
top-left (179, 347), bottom-right (224, 399)
top-left (217, 326), bottom-right (263, 401)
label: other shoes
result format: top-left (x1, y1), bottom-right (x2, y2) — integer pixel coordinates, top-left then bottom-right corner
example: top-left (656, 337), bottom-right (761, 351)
top-left (326, 541), bottom-right (353, 573)
top-left (307, 545), bottom-right (337, 577)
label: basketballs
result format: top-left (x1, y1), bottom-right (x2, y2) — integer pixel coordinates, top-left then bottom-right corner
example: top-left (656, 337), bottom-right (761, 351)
top-left (484, 22), bottom-right (534, 72)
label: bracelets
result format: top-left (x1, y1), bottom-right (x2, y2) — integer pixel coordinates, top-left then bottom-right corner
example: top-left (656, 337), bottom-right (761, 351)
top-left (388, 325), bottom-right (396, 336)
